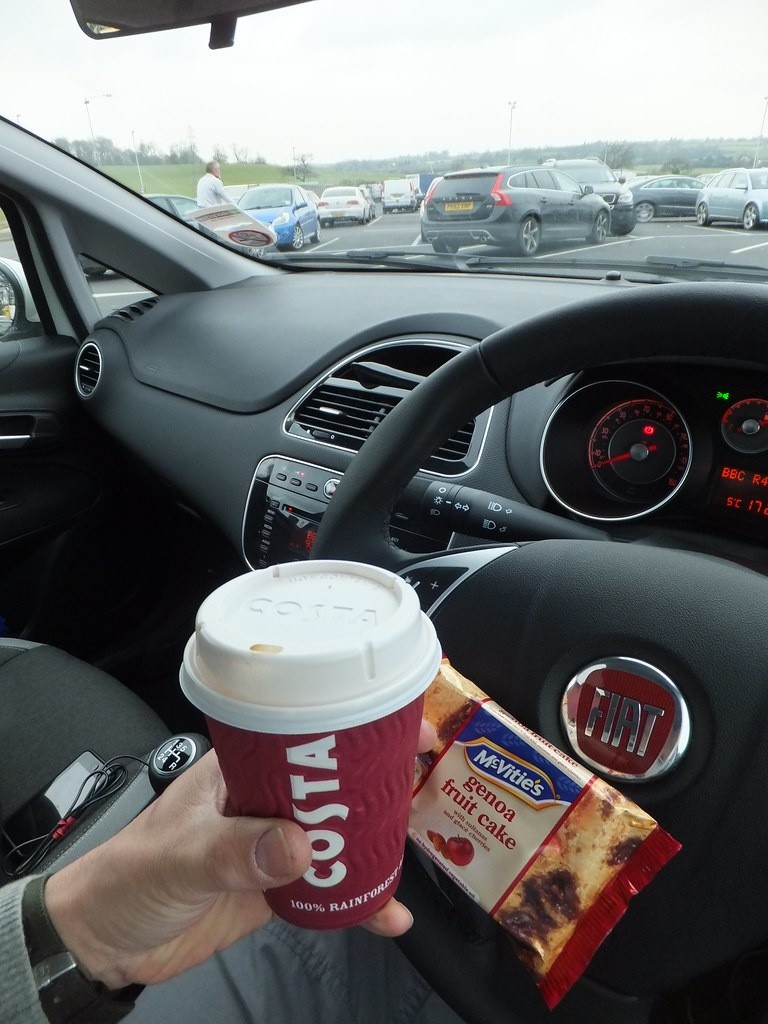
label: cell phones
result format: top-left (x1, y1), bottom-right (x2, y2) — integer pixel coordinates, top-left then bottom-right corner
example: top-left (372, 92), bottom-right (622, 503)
top-left (2, 749), bottom-right (115, 857)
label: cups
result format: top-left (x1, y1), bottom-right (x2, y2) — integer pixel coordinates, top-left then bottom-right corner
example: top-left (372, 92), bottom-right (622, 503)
top-left (179, 559), bottom-right (441, 929)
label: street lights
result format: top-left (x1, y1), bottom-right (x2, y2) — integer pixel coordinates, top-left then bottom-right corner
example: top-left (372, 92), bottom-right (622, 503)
top-left (132, 130), bottom-right (147, 193)
top-left (84, 93), bottom-right (111, 171)
top-left (508, 100), bottom-right (518, 166)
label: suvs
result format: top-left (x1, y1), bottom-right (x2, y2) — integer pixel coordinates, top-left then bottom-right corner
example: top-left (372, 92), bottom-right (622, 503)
top-left (544, 157), bottom-right (637, 235)
top-left (420, 164), bottom-right (611, 257)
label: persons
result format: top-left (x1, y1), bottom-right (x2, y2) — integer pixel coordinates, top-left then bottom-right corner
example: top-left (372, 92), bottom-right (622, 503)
top-left (197, 161), bottom-right (237, 210)
top-left (0, 717), bottom-right (437, 1024)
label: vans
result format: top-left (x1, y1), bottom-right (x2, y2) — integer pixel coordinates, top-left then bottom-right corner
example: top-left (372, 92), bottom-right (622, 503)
top-left (362, 174), bottom-right (419, 214)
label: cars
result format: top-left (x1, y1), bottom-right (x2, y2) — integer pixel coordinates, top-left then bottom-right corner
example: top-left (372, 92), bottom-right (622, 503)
top-left (318, 186), bottom-right (376, 225)
top-left (694, 169), bottom-right (768, 230)
top-left (236, 184), bottom-right (320, 249)
top-left (614, 170), bottom-right (706, 223)
top-left (77, 193), bottom-right (199, 276)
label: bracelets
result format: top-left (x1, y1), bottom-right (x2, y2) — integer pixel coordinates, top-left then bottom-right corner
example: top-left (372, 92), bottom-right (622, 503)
top-left (21, 867), bottom-right (146, 1024)
